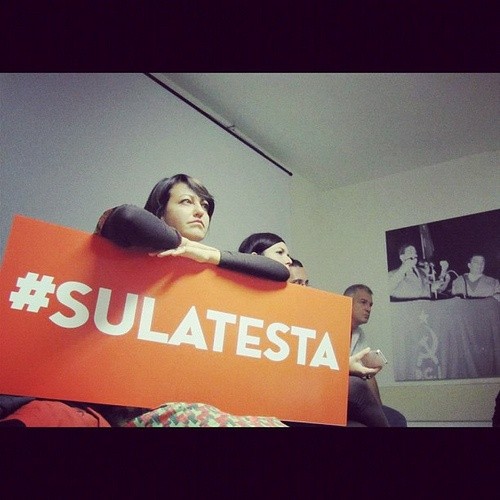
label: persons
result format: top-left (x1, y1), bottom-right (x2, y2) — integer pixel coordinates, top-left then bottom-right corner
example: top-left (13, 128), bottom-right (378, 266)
top-left (287, 259), bottom-right (311, 287)
top-left (344, 284), bottom-right (408, 427)
top-left (452, 255), bottom-right (500, 302)
top-left (388, 242), bottom-right (431, 300)
top-left (238, 232), bottom-right (390, 428)
top-left (0, 175), bottom-right (289, 427)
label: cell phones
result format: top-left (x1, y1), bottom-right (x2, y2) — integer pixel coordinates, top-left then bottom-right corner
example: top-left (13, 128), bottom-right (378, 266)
top-left (360, 350), bottom-right (388, 368)
